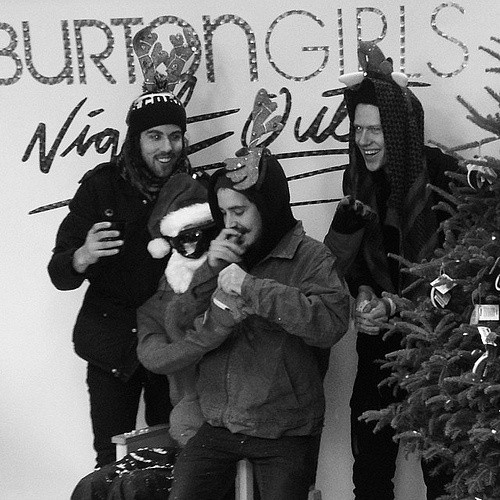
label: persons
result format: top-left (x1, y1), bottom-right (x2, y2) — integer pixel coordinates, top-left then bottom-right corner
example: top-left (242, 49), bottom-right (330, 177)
top-left (140, 144), bottom-right (348, 499)
top-left (49, 89), bottom-right (222, 468)
top-left (319, 80), bottom-right (470, 500)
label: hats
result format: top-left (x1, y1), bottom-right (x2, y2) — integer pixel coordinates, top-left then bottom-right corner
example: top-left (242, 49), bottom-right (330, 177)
top-left (145, 170), bottom-right (210, 258)
top-left (216, 176), bottom-right (254, 203)
top-left (127, 91), bottom-right (187, 136)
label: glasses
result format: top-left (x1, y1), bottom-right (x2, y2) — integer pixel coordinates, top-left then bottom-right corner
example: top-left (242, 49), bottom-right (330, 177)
top-left (159, 221), bottom-right (221, 259)
